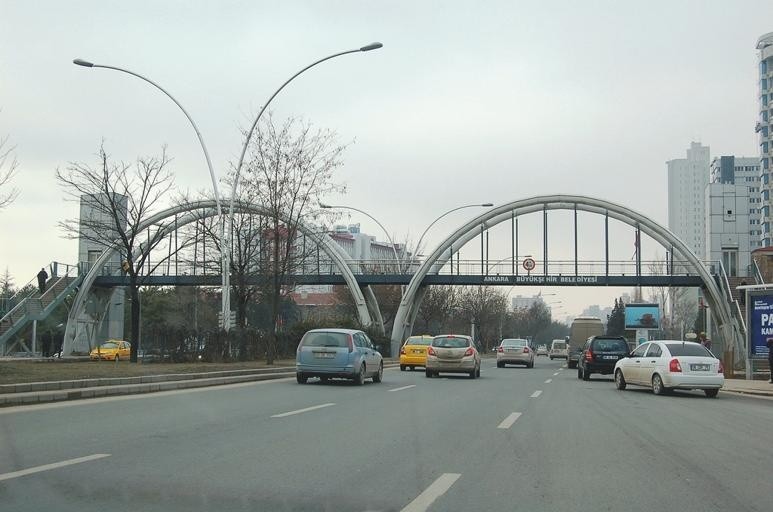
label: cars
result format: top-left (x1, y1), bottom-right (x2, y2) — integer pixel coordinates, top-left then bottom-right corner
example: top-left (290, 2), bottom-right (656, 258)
top-left (494, 338), bottom-right (534, 368)
top-left (88, 337), bottom-right (131, 361)
top-left (397, 335), bottom-right (435, 371)
top-left (611, 338), bottom-right (723, 398)
top-left (424, 333), bottom-right (481, 379)
top-left (536, 346), bottom-right (549, 357)
top-left (491, 346), bottom-right (499, 352)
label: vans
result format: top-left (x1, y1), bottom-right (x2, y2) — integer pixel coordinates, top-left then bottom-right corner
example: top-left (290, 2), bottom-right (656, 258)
top-left (550, 338), bottom-right (568, 360)
top-left (575, 336), bottom-right (630, 379)
top-left (295, 328), bottom-right (384, 385)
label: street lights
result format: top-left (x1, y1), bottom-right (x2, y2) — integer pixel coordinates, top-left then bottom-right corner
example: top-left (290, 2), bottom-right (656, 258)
top-left (69, 40), bottom-right (383, 329)
top-left (513, 293), bottom-right (566, 339)
top-left (319, 199), bottom-right (494, 296)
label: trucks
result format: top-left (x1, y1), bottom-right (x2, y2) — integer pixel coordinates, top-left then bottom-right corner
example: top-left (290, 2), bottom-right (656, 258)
top-left (564, 317), bottom-right (604, 369)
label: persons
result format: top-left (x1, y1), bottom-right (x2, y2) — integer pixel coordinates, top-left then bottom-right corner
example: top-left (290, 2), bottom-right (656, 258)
top-left (699, 332), bottom-right (710, 350)
top-left (766, 336), bottom-right (772, 385)
top-left (739, 278), bottom-right (748, 306)
top-left (37, 267), bottom-right (48, 295)
top-left (120, 258), bottom-right (130, 278)
top-left (52, 330), bottom-right (62, 359)
top-left (41, 330), bottom-right (50, 357)
